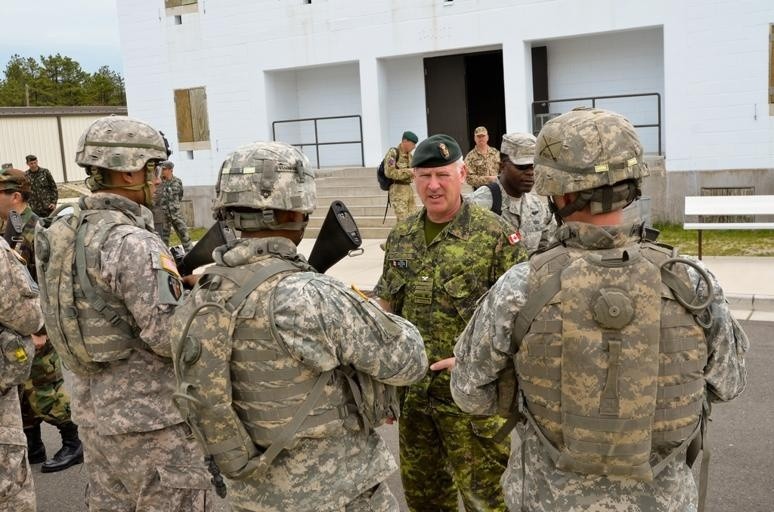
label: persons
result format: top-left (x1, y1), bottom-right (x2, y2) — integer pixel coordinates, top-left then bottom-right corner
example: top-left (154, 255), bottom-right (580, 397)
top-left (384, 125), bottom-right (563, 260)
top-left (174, 141), bottom-right (429, 512)
top-left (1, 153), bottom-right (83, 512)
top-left (449, 106), bottom-right (748, 512)
top-left (366, 134), bottom-right (528, 512)
top-left (32, 116), bottom-right (215, 512)
top-left (147, 158), bottom-right (194, 256)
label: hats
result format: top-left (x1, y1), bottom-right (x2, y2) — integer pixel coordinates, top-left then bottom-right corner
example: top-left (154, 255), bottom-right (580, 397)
top-left (403, 131), bottom-right (419, 143)
top-left (501, 131), bottom-right (539, 166)
top-left (0, 169), bottom-right (35, 195)
top-left (474, 125), bottom-right (487, 137)
top-left (163, 159), bottom-right (174, 169)
top-left (25, 154), bottom-right (38, 162)
top-left (411, 132), bottom-right (462, 169)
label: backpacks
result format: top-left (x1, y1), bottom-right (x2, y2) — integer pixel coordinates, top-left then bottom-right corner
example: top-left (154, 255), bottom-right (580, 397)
top-left (376, 147), bottom-right (400, 190)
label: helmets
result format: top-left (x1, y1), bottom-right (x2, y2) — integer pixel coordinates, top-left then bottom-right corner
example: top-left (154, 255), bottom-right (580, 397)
top-left (212, 138), bottom-right (316, 233)
top-left (72, 113), bottom-right (170, 192)
top-left (532, 102), bottom-right (649, 214)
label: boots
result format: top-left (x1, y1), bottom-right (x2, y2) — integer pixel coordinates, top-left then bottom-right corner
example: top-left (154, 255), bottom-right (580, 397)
top-left (25, 425), bottom-right (48, 464)
top-left (41, 424), bottom-right (85, 474)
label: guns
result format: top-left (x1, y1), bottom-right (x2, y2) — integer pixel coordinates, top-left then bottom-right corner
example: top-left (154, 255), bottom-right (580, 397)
top-left (308, 200), bottom-right (363, 273)
top-left (3, 209), bottom-right (24, 249)
top-left (171, 219), bottom-right (236, 290)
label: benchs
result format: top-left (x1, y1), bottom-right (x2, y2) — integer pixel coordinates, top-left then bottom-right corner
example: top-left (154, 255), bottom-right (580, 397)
top-left (683, 196), bottom-right (774, 261)
